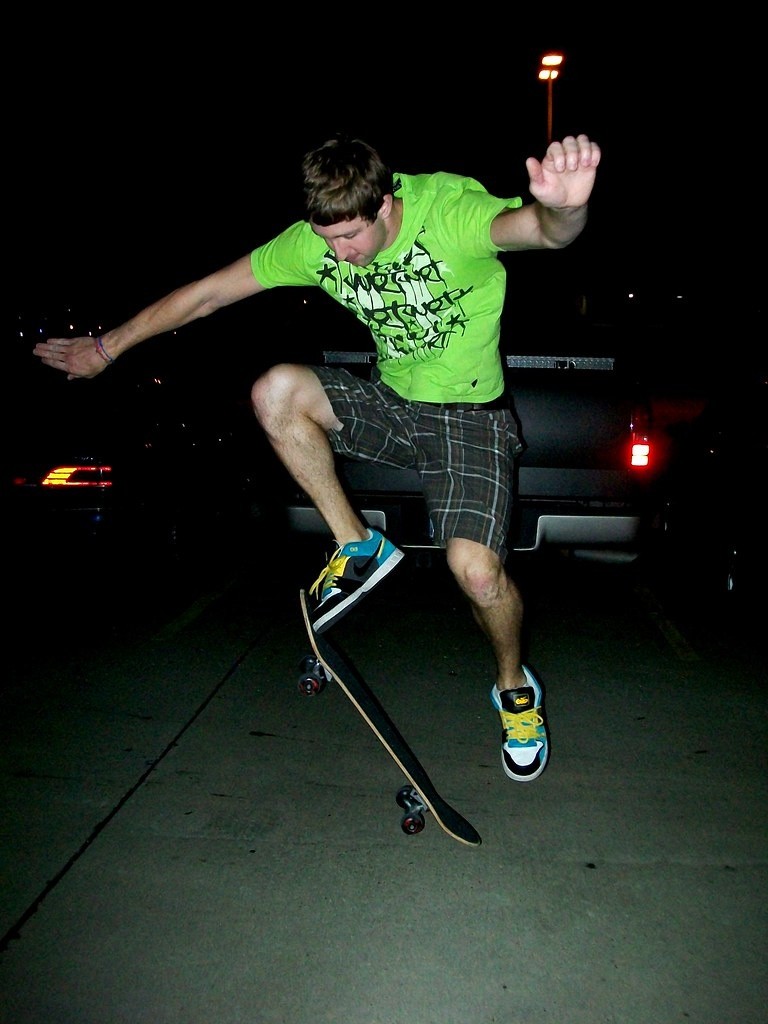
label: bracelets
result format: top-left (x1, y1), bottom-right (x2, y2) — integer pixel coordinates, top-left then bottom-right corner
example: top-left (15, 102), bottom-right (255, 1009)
top-left (95, 337), bottom-right (115, 365)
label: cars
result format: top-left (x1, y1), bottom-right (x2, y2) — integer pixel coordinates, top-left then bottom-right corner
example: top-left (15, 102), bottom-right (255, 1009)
top-left (271, 321), bottom-right (668, 566)
top-left (1, 309), bottom-right (174, 523)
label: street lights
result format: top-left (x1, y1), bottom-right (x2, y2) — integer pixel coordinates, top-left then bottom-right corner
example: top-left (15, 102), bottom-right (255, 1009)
top-left (538, 49), bottom-right (567, 149)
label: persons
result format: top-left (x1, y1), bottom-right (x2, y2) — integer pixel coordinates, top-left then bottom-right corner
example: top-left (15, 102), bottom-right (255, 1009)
top-left (32, 134), bottom-right (603, 781)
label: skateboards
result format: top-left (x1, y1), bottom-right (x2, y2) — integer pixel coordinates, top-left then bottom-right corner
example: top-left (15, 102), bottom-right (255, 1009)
top-left (295, 585), bottom-right (486, 850)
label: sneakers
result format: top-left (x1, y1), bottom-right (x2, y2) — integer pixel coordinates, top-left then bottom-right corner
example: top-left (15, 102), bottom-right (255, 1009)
top-left (490, 665), bottom-right (549, 782)
top-left (309, 526), bottom-right (406, 634)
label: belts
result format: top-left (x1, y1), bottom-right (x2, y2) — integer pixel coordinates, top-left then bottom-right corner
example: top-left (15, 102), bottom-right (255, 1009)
top-left (421, 395), bottom-right (506, 411)
